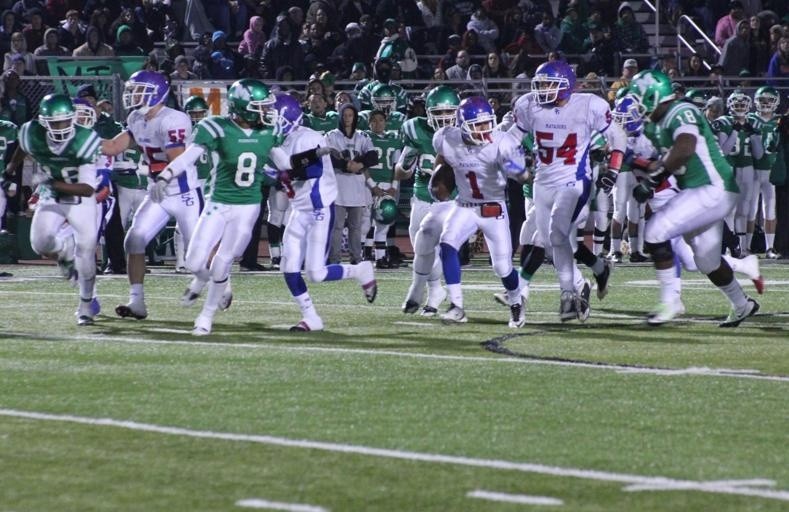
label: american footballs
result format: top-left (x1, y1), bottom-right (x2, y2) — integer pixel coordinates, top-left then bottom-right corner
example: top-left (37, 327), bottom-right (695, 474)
top-left (429, 162), bottom-right (455, 201)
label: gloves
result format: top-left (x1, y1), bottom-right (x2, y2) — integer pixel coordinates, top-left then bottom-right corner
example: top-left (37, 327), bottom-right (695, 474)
top-left (150, 179), bottom-right (166, 202)
top-left (596, 171), bottom-right (619, 195)
top-left (262, 165), bottom-right (281, 180)
top-left (402, 148), bottom-right (420, 170)
top-left (733, 121), bottom-right (753, 132)
top-left (634, 182), bottom-right (654, 202)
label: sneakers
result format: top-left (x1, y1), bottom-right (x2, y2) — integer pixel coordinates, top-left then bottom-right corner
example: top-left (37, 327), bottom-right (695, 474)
top-left (730, 237), bottom-right (761, 259)
top-left (765, 249), bottom-right (785, 261)
top-left (357, 260), bottom-right (376, 303)
top-left (611, 251), bottom-right (647, 263)
top-left (402, 294), bottom-right (527, 328)
top-left (743, 254), bottom-right (764, 294)
top-left (647, 298), bottom-right (685, 326)
top-left (114, 302), bottom-right (149, 320)
top-left (54, 255), bottom-right (100, 325)
top-left (558, 263), bottom-right (610, 321)
top-left (181, 270), bottom-right (233, 336)
top-left (290, 318), bottom-right (324, 332)
top-left (719, 298), bottom-right (760, 327)
top-left (376, 256), bottom-right (408, 268)
top-left (239, 257), bottom-right (280, 270)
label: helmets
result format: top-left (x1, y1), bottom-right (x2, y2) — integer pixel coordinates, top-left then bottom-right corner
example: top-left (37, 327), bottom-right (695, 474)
top-left (227, 79), bottom-right (304, 137)
top-left (184, 96), bottom-right (209, 125)
top-left (373, 191), bottom-right (399, 224)
top-left (531, 61), bottom-right (576, 106)
top-left (371, 83), bottom-right (397, 113)
top-left (424, 86), bottom-right (500, 147)
top-left (686, 87), bottom-right (780, 111)
top-left (122, 70), bottom-right (171, 114)
top-left (38, 93), bottom-right (96, 146)
top-left (610, 68), bottom-right (676, 137)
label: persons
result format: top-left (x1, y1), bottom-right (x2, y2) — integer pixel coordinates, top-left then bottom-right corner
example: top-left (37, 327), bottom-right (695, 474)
top-left (16, 61), bottom-right (764, 339)
top-left (0, 0), bottom-right (789, 310)
